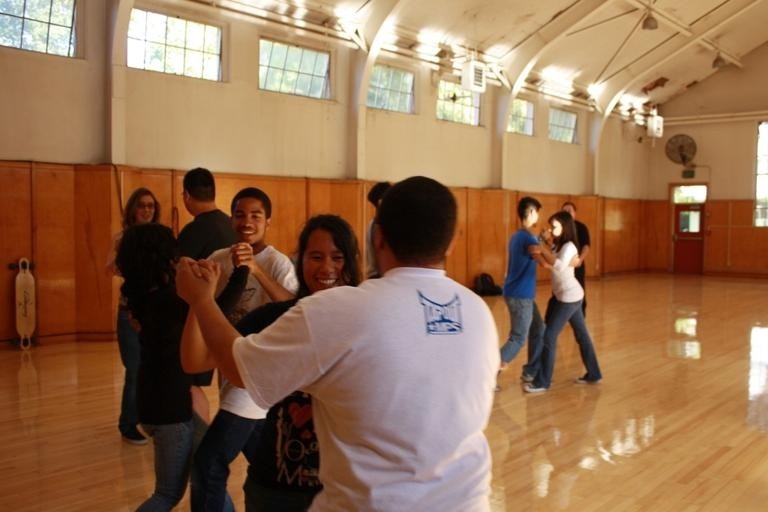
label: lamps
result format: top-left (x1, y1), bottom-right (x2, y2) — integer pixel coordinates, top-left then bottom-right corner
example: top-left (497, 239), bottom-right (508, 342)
top-left (642, 0), bottom-right (658, 32)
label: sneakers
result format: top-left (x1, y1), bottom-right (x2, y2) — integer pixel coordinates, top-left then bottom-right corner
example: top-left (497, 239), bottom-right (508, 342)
top-left (574, 376), bottom-right (603, 384)
top-left (117, 423), bottom-right (148, 446)
top-left (494, 366), bottom-right (553, 393)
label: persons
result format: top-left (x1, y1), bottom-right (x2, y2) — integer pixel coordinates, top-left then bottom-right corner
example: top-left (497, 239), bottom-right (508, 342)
top-left (176, 176), bottom-right (500, 512)
top-left (363, 183), bottom-right (394, 280)
top-left (524, 210), bottom-right (604, 394)
top-left (176, 214), bottom-right (360, 512)
top-left (495, 197), bottom-right (546, 392)
top-left (175, 167), bottom-right (237, 257)
top-left (118, 247), bottom-right (255, 512)
top-left (541, 203), bottom-right (592, 331)
top-left (115, 189), bottom-right (178, 446)
top-left (187, 185), bottom-right (301, 512)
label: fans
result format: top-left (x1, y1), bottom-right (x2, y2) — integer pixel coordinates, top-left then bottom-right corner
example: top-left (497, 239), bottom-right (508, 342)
top-left (664, 131), bottom-right (698, 171)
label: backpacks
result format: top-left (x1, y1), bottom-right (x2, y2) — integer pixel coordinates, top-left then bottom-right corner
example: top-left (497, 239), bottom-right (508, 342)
top-left (473, 273), bottom-right (502, 296)
